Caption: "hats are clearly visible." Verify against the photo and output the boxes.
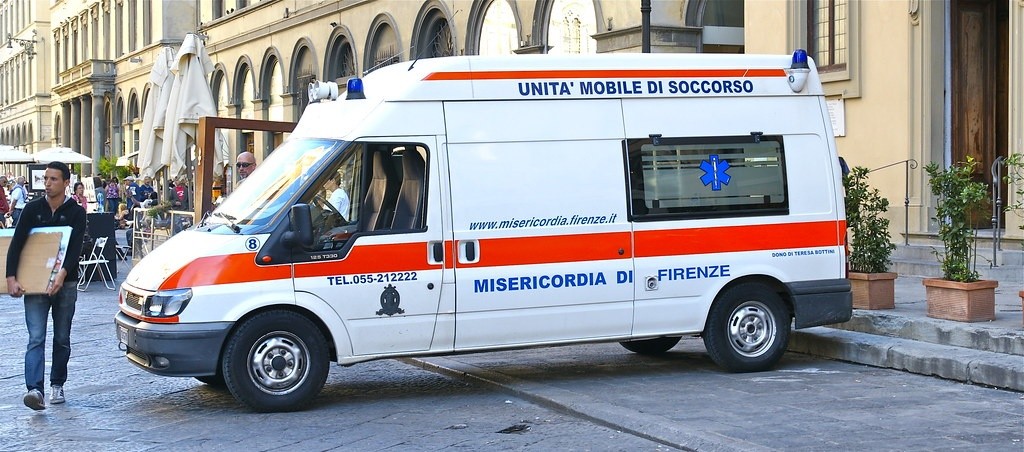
[123,176,134,180]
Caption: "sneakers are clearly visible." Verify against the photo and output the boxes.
[49,384,65,404]
[24,389,45,410]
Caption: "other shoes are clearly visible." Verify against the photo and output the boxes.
[123,252,132,256]
[119,255,128,261]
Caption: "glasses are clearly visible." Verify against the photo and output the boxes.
[236,162,255,168]
[2,181,8,183]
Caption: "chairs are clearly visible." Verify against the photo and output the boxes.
[76,237,116,291]
[358,149,400,231]
[389,147,425,231]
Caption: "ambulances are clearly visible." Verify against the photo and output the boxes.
[114,50,855,415]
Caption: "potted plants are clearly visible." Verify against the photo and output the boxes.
[999,153,1023,331]
[842,165,899,310]
[147,200,173,227]
[923,154,999,323]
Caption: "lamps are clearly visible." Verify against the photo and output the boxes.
[7,33,37,61]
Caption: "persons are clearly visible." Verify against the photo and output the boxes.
[4,159,87,410]
[70,173,193,232]
[0,175,28,229]
[322,172,350,224]
[234,150,257,191]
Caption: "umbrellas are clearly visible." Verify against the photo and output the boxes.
[159,33,226,213]
[136,44,178,206]
[29,143,94,165]
[0,147,36,163]
